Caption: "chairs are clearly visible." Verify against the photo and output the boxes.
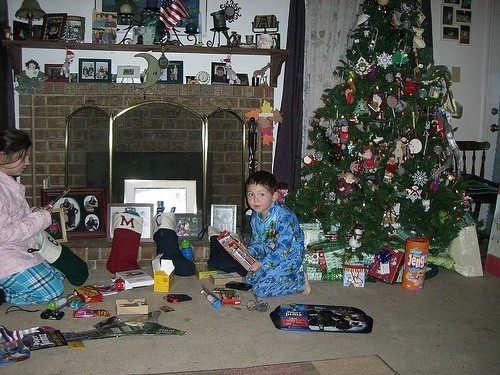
[455,141,499,273]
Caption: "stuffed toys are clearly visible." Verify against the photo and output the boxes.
[60,51,74,77]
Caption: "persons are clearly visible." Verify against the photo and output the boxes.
[49,64,108,78]
[0,127,64,308]
[172,65,178,80]
[46,24,74,39]
[92,13,116,44]
[25,60,39,78]
[50,196,99,235]
[215,66,226,81]
[240,170,311,298]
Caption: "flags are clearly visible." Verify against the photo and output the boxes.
[159,0,190,30]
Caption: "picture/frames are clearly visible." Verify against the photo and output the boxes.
[211,62,227,84]
[175,213,202,240]
[441,0,473,45]
[41,188,107,238]
[45,64,69,82]
[117,66,139,78]
[256,33,280,49]
[167,61,183,84]
[124,179,197,216]
[107,203,153,243]
[211,204,236,233]
[13,13,85,41]
[44,208,67,243]
[79,59,111,83]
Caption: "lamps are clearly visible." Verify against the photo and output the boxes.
[15,0,46,40]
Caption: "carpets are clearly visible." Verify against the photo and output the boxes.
[133,354,401,375]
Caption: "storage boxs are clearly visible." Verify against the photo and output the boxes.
[196,231,257,285]
[270,304,373,333]
[300,222,405,288]
[74,253,175,315]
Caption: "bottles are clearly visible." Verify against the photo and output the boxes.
[46,297,68,310]
[181,239,194,261]
